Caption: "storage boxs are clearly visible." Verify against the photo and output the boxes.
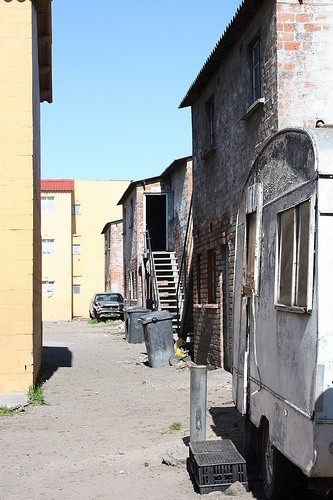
[185,437,251,495]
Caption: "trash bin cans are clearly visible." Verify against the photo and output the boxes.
[136,310,175,368]
[122,306,149,344]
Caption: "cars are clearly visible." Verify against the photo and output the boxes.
[89,292,125,323]
